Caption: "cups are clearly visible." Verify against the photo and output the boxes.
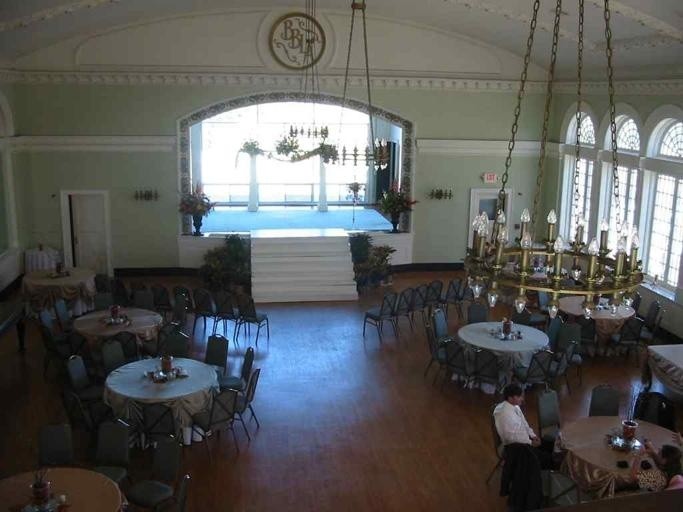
[21,481,70,512]
[143,357,186,387]
[487,322,523,341]
[100,302,130,328]
[602,417,642,457]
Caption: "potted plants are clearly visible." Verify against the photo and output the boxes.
[349,232,397,294]
[197,234,251,293]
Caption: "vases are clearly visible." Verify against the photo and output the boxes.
[192,214,204,236]
[389,211,401,233]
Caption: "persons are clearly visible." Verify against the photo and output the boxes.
[492,384,563,471]
[631,428,682,495]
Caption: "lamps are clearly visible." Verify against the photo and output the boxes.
[324,0,389,171]
[460,0,646,319]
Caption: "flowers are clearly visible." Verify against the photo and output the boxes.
[178,183,216,217]
[379,188,418,214]
